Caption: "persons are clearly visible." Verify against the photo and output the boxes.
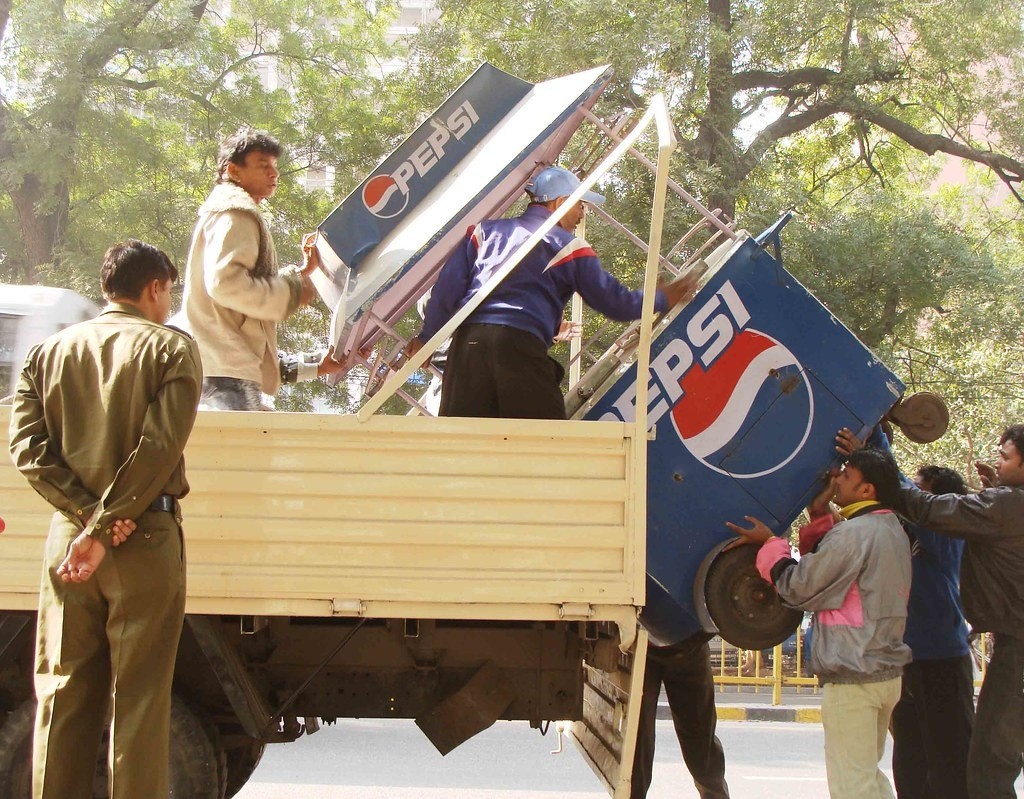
[164,128,373,412]
[632,641,732,799]
[721,420,1024,799]
[6,238,204,796]
[406,165,701,420]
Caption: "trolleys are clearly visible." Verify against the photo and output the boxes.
[310,62,949,650]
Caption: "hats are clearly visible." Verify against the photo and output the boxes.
[525,167,606,204]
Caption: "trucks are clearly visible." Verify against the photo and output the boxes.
[0,93,676,799]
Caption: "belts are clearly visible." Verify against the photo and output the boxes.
[147,495,175,512]
